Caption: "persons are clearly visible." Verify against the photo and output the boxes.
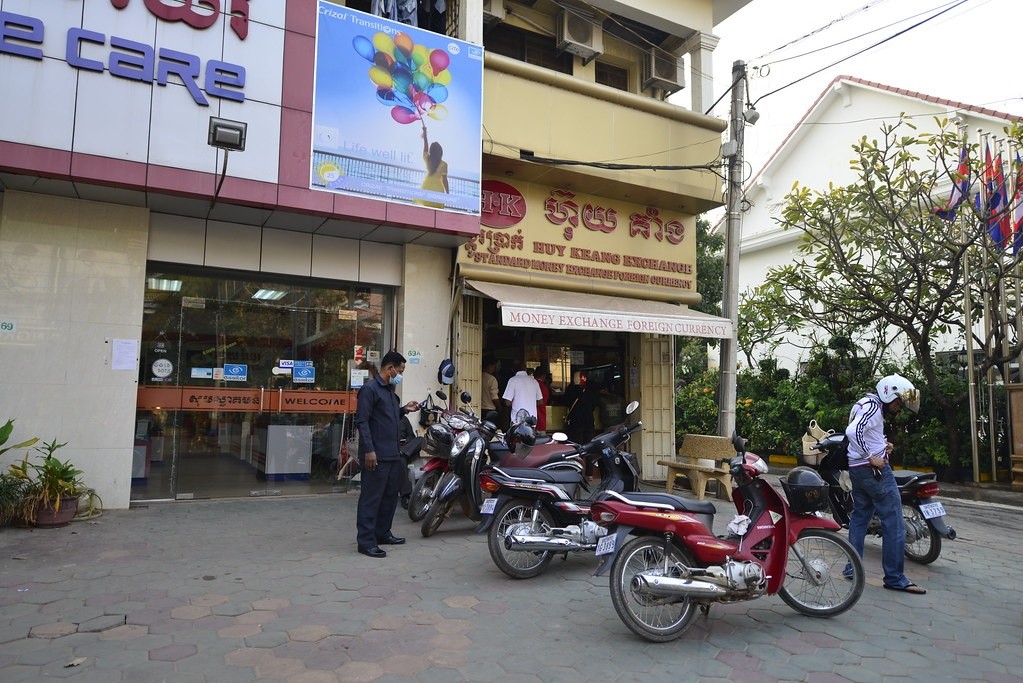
[415,126,450,210]
[841,374,926,594]
[480,355,552,435]
[356,352,427,558]
[598,382,628,451]
[562,370,597,481]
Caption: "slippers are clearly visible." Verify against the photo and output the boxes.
[848,576,853,580]
[883,583,926,594]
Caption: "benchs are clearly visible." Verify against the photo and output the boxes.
[657,435,748,502]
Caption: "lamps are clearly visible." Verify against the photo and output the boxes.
[208,117,248,213]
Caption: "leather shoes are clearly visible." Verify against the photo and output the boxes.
[358,545,386,557]
[378,535,406,544]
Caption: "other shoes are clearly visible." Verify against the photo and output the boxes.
[401,495,410,509]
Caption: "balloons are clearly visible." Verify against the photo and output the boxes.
[352,31,452,124]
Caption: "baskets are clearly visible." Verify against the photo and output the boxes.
[592,451,640,476]
[797,451,829,470]
[421,434,451,459]
[779,477,830,512]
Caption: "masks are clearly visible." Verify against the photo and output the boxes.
[389,367,403,385]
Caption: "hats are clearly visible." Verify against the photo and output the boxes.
[441,359,455,385]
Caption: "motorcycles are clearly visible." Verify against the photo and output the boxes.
[806,425,956,565]
[408,390,555,522]
[588,436,865,643]
[472,401,647,578]
[421,392,591,537]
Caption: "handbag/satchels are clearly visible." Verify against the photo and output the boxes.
[802,420,835,465]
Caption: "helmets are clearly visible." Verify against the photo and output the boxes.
[426,423,453,449]
[875,372,915,404]
[506,423,537,453]
[785,466,825,508]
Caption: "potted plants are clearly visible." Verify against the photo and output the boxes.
[19,437,103,527]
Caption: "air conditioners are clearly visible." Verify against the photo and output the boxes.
[556,10,604,63]
[483,0,505,25]
[643,48,686,94]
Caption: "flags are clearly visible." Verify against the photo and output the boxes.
[928,132,1023,254]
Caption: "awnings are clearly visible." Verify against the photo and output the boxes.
[466,279,733,340]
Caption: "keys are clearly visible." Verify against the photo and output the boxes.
[872,467,883,480]
